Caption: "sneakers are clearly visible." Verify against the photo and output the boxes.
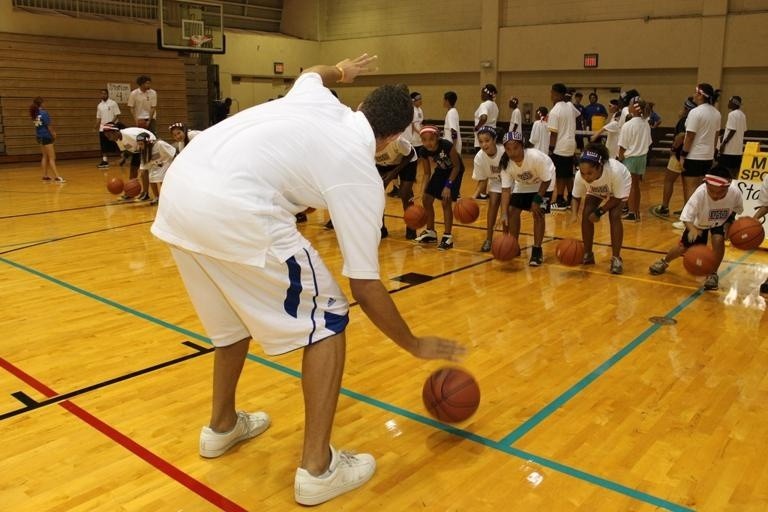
[566,201,572,209]
[382,227,388,237]
[436,235,454,251]
[549,199,567,210]
[760,278,768,294]
[648,257,670,276]
[293,443,378,507]
[198,410,271,459]
[120,159,125,166]
[40,176,54,182]
[703,271,719,290]
[55,177,66,182]
[515,246,521,257]
[296,213,307,223]
[414,229,437,243]
[655,206,670,216]
[622,209,628,213]
[621,213,636,222]
[386,185,401,198]
[609,256,624,274]
[528,246,543,266]
[481,240,492,252]
[97,160,109,167]
[117,192,158,206]
[322,219,333,230]
[671,220,686,230]
[476,192,488,201]
[406,226,417,240]
[582,251,595,265]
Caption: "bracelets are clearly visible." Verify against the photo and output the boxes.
[336,65,344,83]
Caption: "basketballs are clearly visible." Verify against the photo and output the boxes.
[454,197,479,223]
[729,216,764,249]
[492,234,518,261]
[556,239,584,265]
[684,245,716,276]
[404,204,427,229]
[125,180,141,197]
[422,369,480,423]
[107,178,124,194]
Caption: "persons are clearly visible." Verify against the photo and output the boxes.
[29,97,65,184]
[650,83,767,294]
[217,97,232,122]
[95,75,205,205]
[328,82,660,275]
[150,53,465,508]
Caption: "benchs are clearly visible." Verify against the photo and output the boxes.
[417,114,768,166]
